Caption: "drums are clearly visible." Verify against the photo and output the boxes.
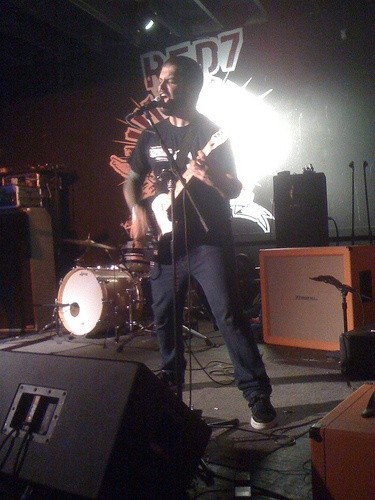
[119,240,154,271]
[56,264,145,337]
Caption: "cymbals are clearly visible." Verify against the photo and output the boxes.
[59,236,118,251]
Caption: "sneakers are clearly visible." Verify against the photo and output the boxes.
[249,399,276,429]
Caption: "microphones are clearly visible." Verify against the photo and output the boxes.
[128,95,166,119]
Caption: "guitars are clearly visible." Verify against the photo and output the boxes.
[148,127,230,243]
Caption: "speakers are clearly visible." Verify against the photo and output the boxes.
[0,351,212,500]
[272,172,329,248]
[309,383,375,500]
[259,244,375,350]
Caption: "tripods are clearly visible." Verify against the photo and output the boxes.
[118,288,144,332]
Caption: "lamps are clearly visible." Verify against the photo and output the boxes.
[139,16,156,31]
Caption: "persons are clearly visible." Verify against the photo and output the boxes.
[123,56,278,430]
